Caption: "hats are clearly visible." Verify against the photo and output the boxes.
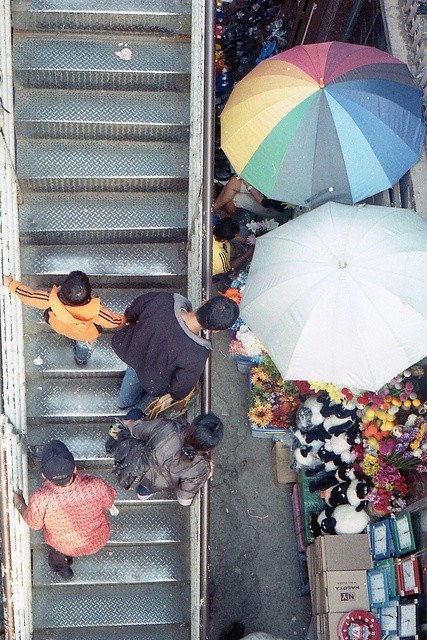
[41,440,74,484]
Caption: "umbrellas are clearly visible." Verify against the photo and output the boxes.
[238,201,426,396]
[218,40,426,209]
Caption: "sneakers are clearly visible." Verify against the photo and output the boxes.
[136,492,159,500]
[220,621,244,640]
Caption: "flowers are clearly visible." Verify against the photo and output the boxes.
[224,272,248,305]
[229,326,266,360]
[248,360,310,429]
[246,219,279,246]
[312,364,426,504]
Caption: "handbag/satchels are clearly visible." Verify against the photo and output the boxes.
[113,438,153,491]
[142,386,199,420]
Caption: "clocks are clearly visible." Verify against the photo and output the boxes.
[381,595,400,635]
[398,605,421,637]
[367,570,389,608]
[337,610,381,640]
[400,555,422,596]
[389,520,399,557]
[371,520,390,560]
[375,559,396,598]
[394,512,417,554]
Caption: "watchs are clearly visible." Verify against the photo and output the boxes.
[16,499,24,511]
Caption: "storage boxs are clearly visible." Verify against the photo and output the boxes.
[292,483,307,553]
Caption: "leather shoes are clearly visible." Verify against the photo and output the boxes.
[59,566,73,581]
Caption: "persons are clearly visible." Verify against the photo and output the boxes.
[213,176,290,218]
[121,408,223,506]
[112,291,239,413]
[3,271,126,365]
[220,621,275,639]
[13,440,117,581]
[213,217,255,293]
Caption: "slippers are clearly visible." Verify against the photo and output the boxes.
[254,40,275,64]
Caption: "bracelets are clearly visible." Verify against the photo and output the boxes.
[248,186,252,191]
[241,254,248,260]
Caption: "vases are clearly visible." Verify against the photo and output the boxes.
[248,355,311,440]
[236,356,258,374]
